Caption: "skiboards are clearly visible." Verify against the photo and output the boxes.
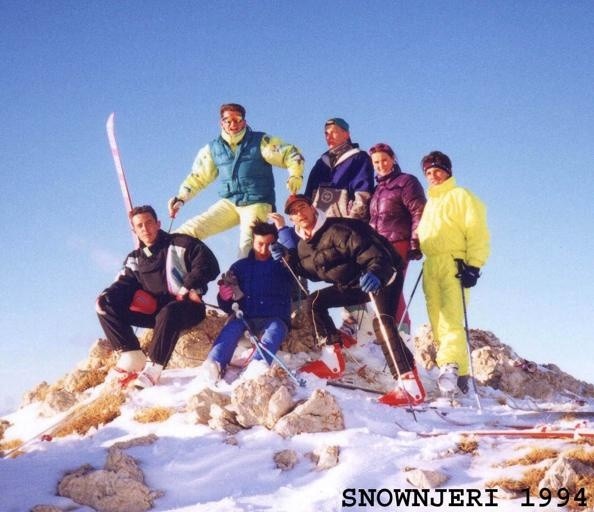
[395,400,594,439]
[105,111,140,251]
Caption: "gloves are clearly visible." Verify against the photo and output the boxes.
[406,249,423,260]
[218,270,244,301]
[359,271,382,296]
[168,194,187,217]
[176,285,190,302]
[286,176,304,195]
[268,242,291,263]
[95,288,115,316]
[462,266,481,288]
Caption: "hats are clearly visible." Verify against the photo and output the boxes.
[423,151,452,176]
[324,117,352,145]
[284,193,313,215]
[370,142,395,161]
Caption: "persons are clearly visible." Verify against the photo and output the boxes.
[282,194,427,408]
[164,103,306,260]
[301,118,375,348]
[411,151,490,396]
[93,204,221,392]
[362,142,425,335]
[190,210,302,386]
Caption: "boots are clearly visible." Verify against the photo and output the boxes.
[295,343,347,384]
[351,305,378,338]
[379,367,425,408]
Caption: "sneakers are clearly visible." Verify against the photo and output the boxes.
[231,360,272,391]
[192,360,227,391]
[100,366,153,389]
[437,361,458,393]
